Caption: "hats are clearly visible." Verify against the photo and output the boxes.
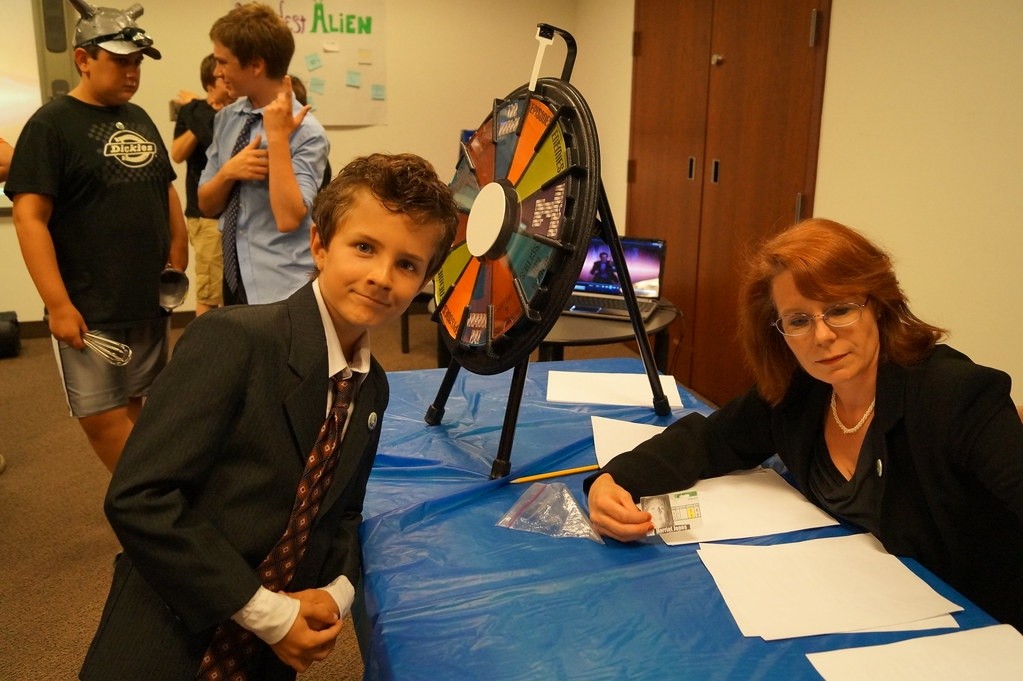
[70,0,162,62]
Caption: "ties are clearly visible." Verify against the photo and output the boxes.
[197,364,357,681]
[222,114,260,293]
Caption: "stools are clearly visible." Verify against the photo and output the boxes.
[401,280,435,354]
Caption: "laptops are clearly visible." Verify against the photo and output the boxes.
[561,236,666,320]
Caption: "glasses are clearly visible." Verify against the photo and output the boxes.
[769,292,870,336]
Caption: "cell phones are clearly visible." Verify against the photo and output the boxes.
[570,304,602,313]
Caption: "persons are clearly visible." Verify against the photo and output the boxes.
[584,219,1023,631]
[2,0,189,471]
[590,253,619,285]
[171,55,237,316]
[640,495,674,529]
[197,3,330,306]
[290,76,332,190]
[0,138,16,473]
[76,153,460,681]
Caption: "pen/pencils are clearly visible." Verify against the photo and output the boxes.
[510,465,599,483]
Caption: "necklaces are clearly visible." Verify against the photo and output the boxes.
[831,390,876,433]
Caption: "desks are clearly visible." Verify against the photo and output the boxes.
[352,358,997,681]
[534,293,678,375]
